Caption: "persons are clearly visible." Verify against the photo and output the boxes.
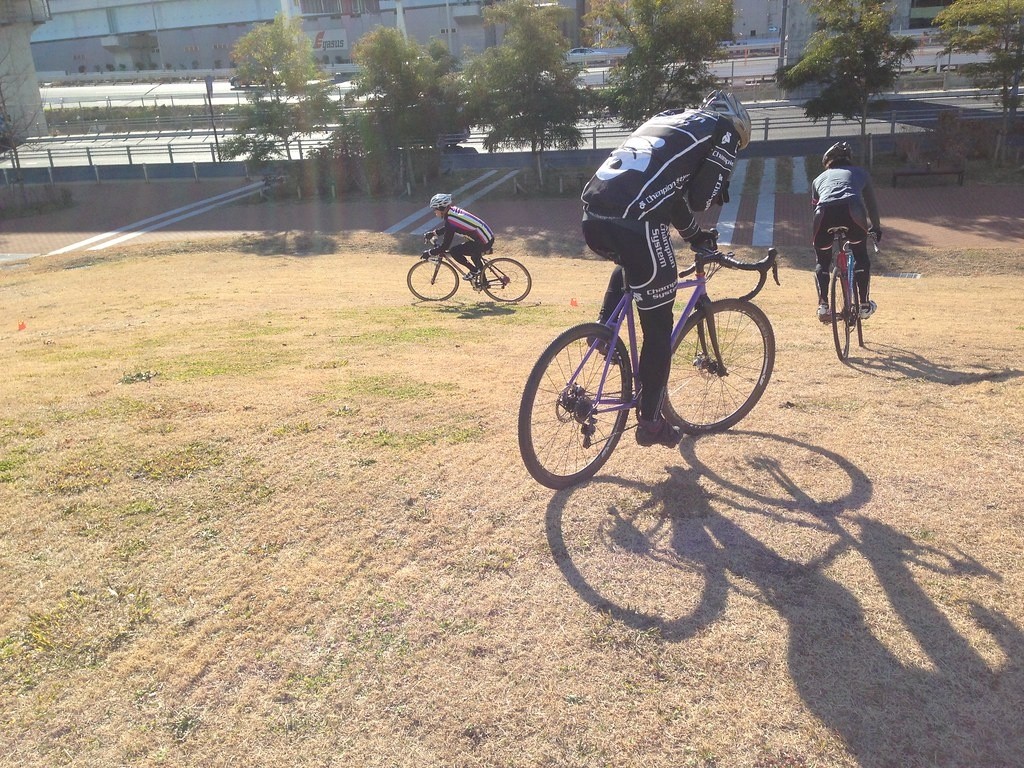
[420,193,495,289]
[580,88,753,449]
[811,142,883,322]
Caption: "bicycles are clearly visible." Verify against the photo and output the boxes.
[407,232,532,305]
[515,233,779,490]
[829,224,887,362]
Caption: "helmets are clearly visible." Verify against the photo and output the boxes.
[699,89,751,150]
[822,141,852,166]
[430,193,452,209]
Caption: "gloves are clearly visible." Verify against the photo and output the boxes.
[420,250,430,259]
[423,231,434,244]
[684,228,717,252]
[867,226,882,242]
[717,181,730,207]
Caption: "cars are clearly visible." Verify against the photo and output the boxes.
[565,46,608,66]
[229,68,281,90]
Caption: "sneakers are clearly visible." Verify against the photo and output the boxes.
[586,320,618,365]
[636,416,683,449]
[817,303,830,322]
[859,300,878,319]
[462,269,481,280]
